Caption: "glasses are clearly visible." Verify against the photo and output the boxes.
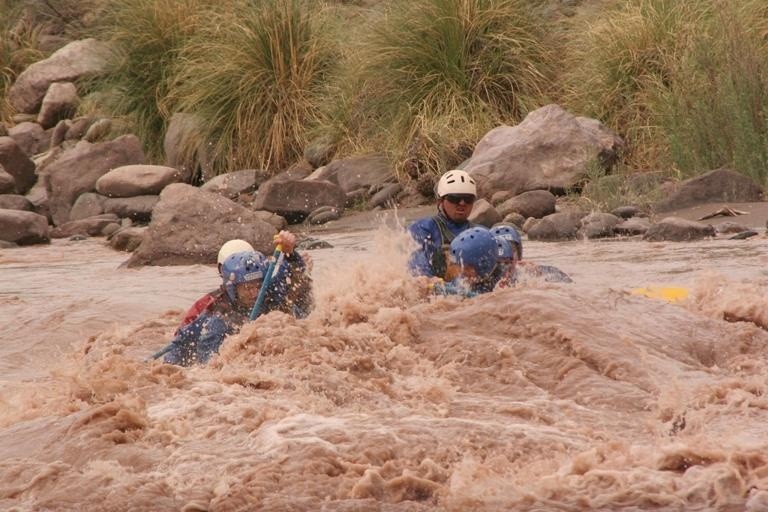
[444,194,475,204]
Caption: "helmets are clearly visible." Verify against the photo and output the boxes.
[448,225,522,278]
[437,170,477,199]
[217,239,270,303]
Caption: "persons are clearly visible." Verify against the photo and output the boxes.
[404,167,572,298]
[163,230,312,370]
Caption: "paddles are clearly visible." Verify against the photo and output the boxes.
[631,286,693,303]
